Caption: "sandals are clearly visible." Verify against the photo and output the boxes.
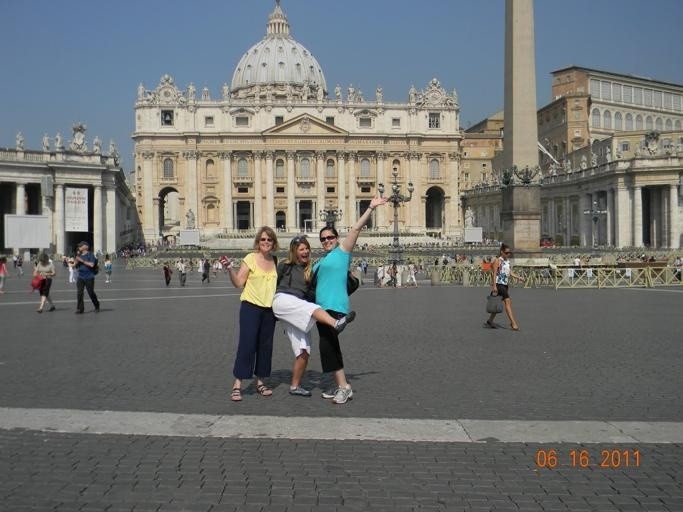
[230,383,242,401]
[255,383,272,396]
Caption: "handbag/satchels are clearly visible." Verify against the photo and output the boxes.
[486,291,503,313]
[91,259,98,275]
[30,275,43,289]
[305,269,359,301]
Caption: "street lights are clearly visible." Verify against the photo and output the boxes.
[375,165,414,261]
[583,199,608,247]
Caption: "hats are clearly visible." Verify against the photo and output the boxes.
[75,241,88,246]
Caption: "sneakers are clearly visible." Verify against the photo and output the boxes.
[35,308,42,313]
[510,323,520,331]
[321,379,353,404]
[45,305,55,311]
[483,318,499,330]
[73,308,84,314]
[94,306,99,313]
[330,311,355,334]
[288,384,311,396]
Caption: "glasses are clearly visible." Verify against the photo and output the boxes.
[502,251,512,255]
[291,236,306,246]
[319,236,336,242]
[259,238,272,242]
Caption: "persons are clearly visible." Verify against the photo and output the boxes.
[137,84,147,102]
[14,133,26,149]
[222,85,230,101]
[68,241,100,314]
[42,134,62,149]
[0,241,683,294]
[252,85,384,105]
[33,254,56,313]
[550,146,645,175]
[409,86,419,106]
[272,235,356,396]
[185,85,196,100]
[94,138,103,151]
[219,226,280,401]
[486,243,525,331]
[309,195,388,404]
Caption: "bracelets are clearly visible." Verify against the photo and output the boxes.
[368,205,374,210]
[518,277,521,281]
